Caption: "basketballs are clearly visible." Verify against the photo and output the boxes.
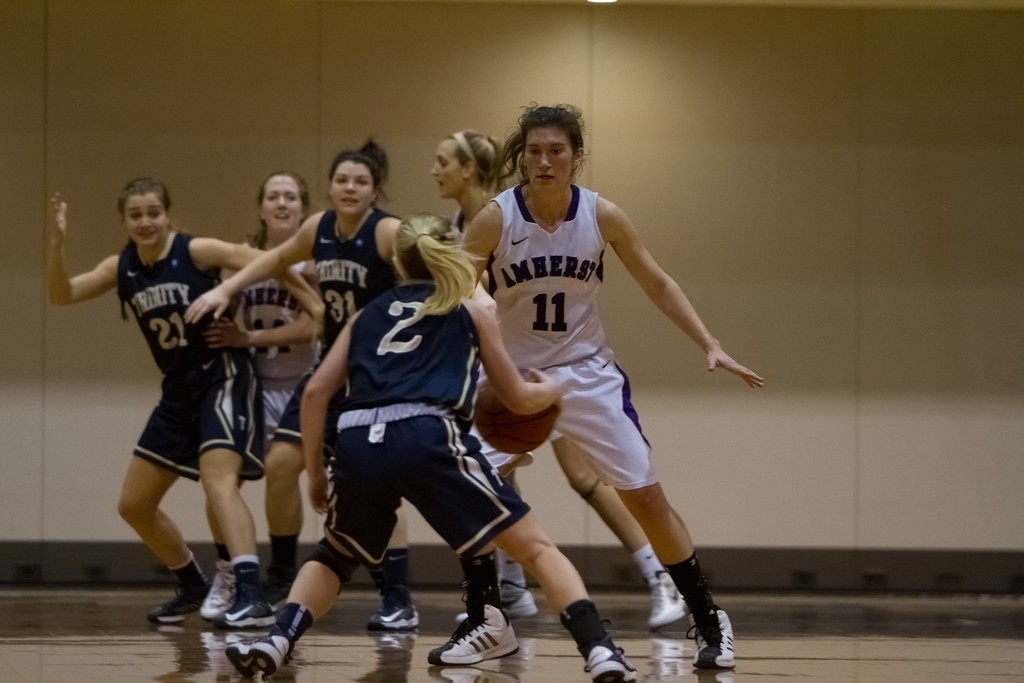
[473,369,559,453]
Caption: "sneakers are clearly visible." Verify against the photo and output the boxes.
[366,584,419,631]
[647,570,690,630]
[147,550,211,624]
[428,665,520,683]
[686,605,736,669]
[225,625,290,681]
[224,562,277,629]
[428,580,520,665]
[260,566,295,613]
[455,577,538,622]
[200,558,237,622]
[577,631,639,683]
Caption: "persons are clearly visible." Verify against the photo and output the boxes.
[425,107,765,671]
[31,176,326,635]
[198,139,638,683]
[428,128,692,631]
